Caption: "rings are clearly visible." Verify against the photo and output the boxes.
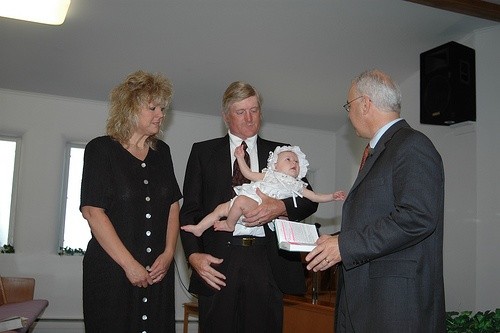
[325,259,330,263]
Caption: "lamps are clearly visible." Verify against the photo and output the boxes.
[0,0,73,25]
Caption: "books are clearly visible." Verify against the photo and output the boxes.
[273,219,319,252]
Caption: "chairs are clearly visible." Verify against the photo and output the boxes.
[0,276,48,333]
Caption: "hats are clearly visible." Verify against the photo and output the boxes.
[267,145,309,180]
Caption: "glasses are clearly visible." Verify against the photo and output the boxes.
[344,95,371,112]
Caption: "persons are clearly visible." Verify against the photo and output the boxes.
[306,71,448,333]
[181,144,346,238]
[179,82,318,333]
[79,71,183,333]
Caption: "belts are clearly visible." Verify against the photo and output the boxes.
[234,237,266,246]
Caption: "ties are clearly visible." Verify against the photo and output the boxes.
[359,142,370,173]
[232,141,251,185]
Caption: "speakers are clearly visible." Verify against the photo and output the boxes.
[420,41,476,125]
